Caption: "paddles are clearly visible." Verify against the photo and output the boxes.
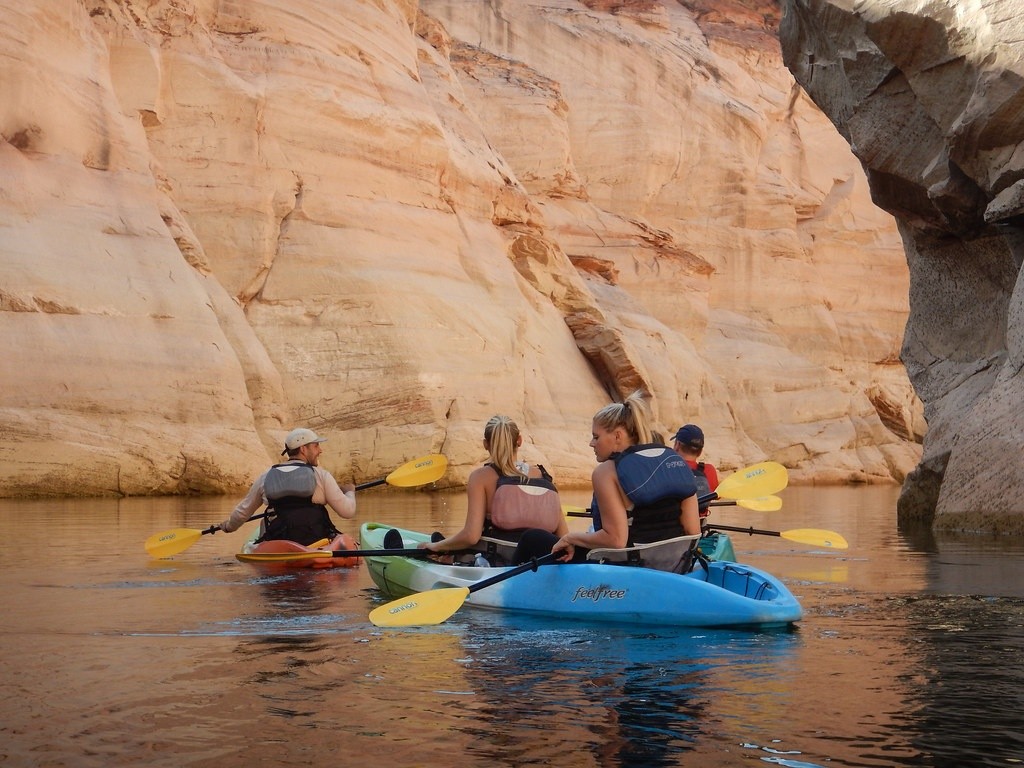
[143,453,450,560]
[560,494,784,520]
[368,458,790,628]
[234,547,476,563]
[560,503,850,551]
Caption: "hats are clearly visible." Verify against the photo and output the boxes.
[281,428,329,456]
[670,424,705,448]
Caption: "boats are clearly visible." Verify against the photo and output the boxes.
[236,519,366,567]
[360,521,804,631]
[695,516,736,562]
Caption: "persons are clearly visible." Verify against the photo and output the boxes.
[669,424,718,526]
[420,416,568,566]
[218,427,356,548]
[550,391,701,563]
